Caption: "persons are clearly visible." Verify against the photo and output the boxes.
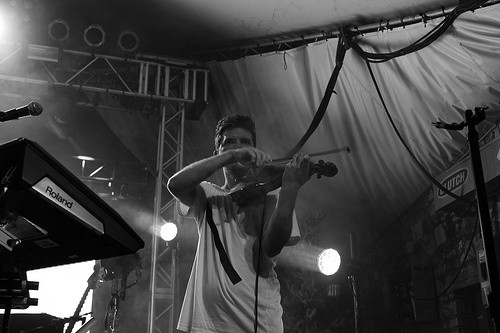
[165,114,317,333]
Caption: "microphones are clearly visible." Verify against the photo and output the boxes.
[0,100,43,122]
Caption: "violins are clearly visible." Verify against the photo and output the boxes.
[230,155,338,207]
